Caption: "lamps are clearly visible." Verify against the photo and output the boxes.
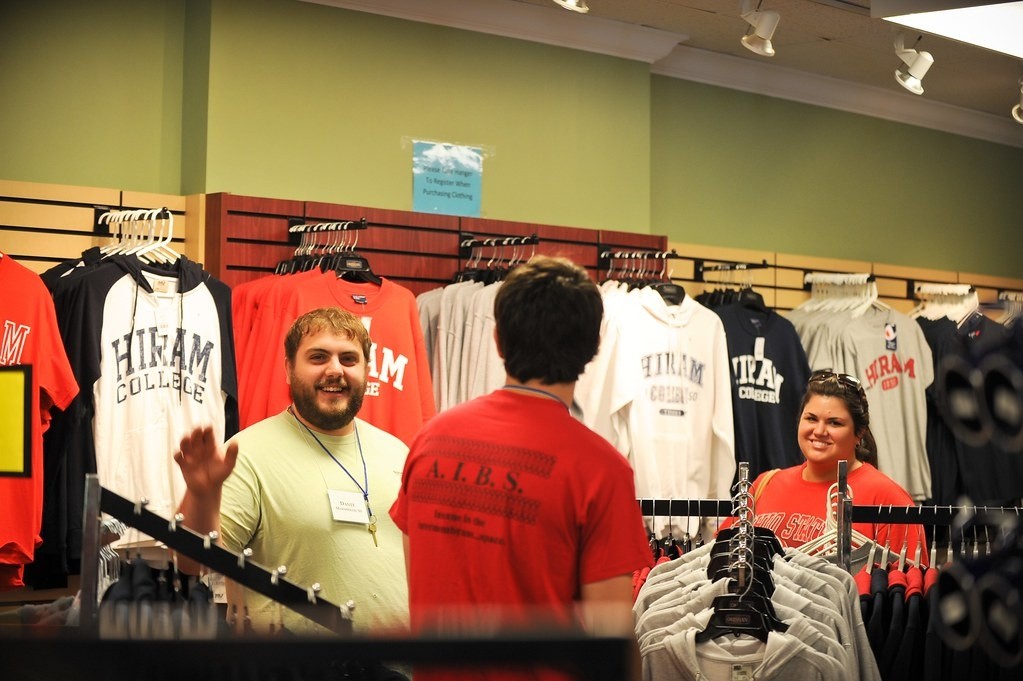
[552,0,590,14]
[893,30,935,95]
[740,0,781,57]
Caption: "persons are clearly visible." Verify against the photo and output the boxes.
[713,371,931,570]
[386,254,656,681]
[166,307,412,639]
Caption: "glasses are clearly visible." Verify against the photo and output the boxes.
[809,371,864,401]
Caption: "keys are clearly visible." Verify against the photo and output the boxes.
[369,515,378,547]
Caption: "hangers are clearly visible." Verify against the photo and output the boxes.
[60,207,183,278]
[66,518,297,638]
[995,290,1023,328]
[797,272,891,317]
[597,250,686,306]
[443,236,536,286]
[693,262,767,313]
[270,220,382,288]
[907,282,983,329]
[637,477,1023,644]
